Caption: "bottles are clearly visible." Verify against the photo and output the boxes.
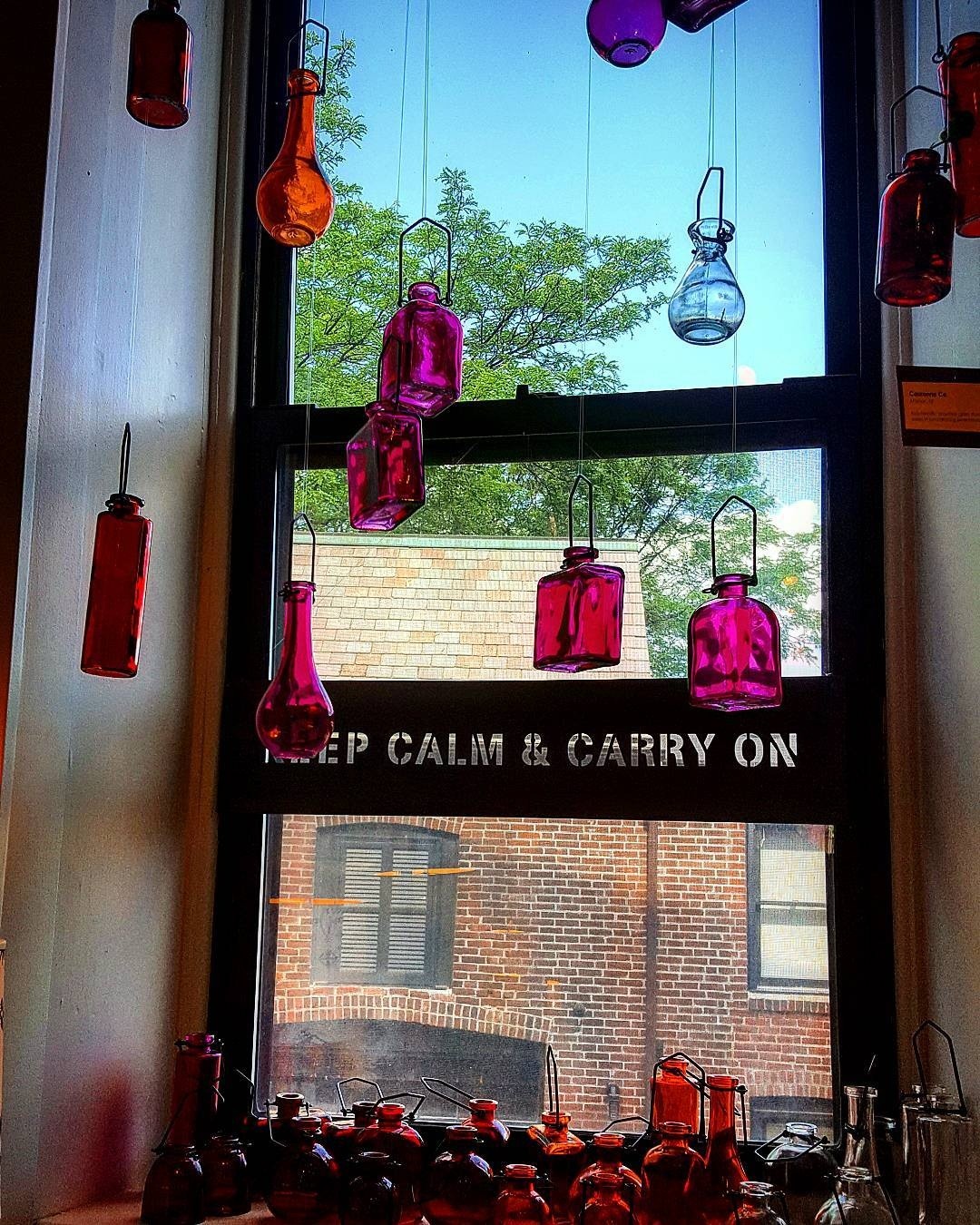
[381,280,464,419]
[686,573,783,712]
[667,217,746,345]
[873,148,958,308]
[938,32,980,240]
[80,493,154,679]
[255,68,337,249]
[125,0,197,129]
[255,580,336,759]
[141,1017,980,1225]
[533,547,626,674]
[346,401,427,532]
[585,0,748,69]
